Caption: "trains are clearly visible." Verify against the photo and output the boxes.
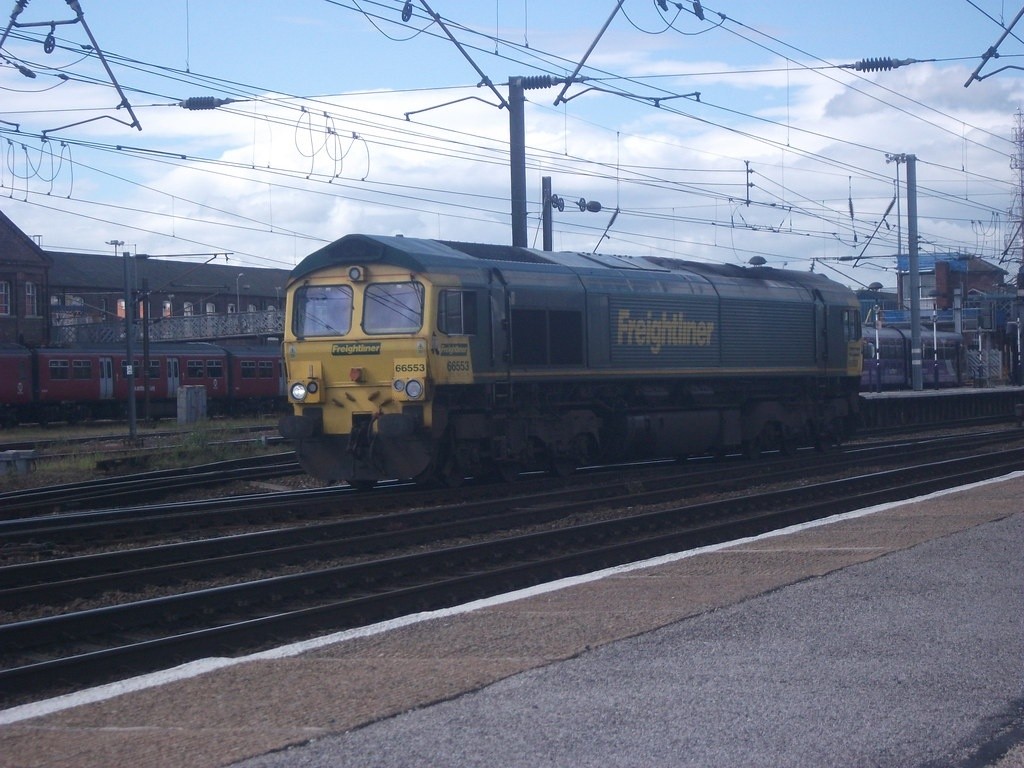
[267,230,866,472]
[1,320,970,419]
